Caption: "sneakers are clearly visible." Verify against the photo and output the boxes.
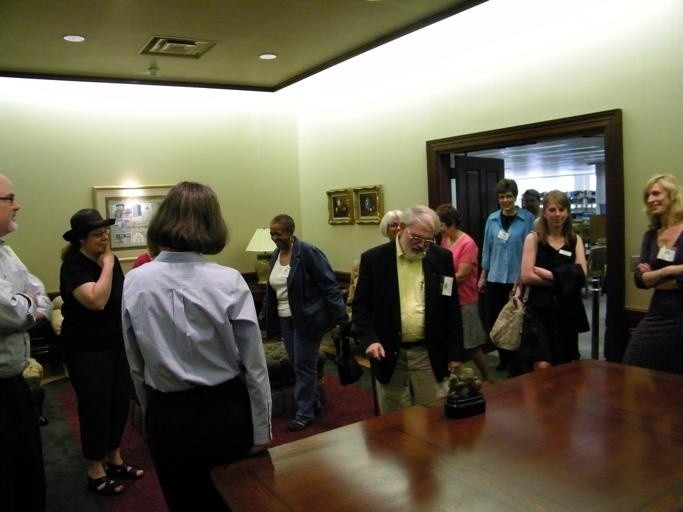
[287,415,313,432]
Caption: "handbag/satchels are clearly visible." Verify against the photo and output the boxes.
[334,337,363,386]
[489,293,528,350]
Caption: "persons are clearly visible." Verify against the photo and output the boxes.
[623,174,683,377]
[379,210,404,243]
[121,182,273,512]
[361,206,465,416]
[477,179,538,372]
[59,209,145,495]
[1,175,55,512]
[436,203,494,384]
[521,189,587,373]
[258,213,348,432]
[524,189,543,220]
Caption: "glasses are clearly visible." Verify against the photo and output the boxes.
[407,225,436,248]
[88,229,110,238]
[1,195,15,203]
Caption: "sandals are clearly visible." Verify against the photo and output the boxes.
[104,457,145,480]
[87,476,126,494]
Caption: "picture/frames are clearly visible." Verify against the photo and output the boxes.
[326,188,354,225]
[352,184,383,225]
[92,184,177,262]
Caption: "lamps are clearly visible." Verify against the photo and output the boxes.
[245,229,277,285]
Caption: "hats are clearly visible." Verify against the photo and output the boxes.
[64,209,116,241]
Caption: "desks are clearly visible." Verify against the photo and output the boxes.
[209,359,683,512]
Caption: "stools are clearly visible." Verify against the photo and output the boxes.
[263,341,327,419]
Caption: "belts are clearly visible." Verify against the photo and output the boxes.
[400,341,425,350]
